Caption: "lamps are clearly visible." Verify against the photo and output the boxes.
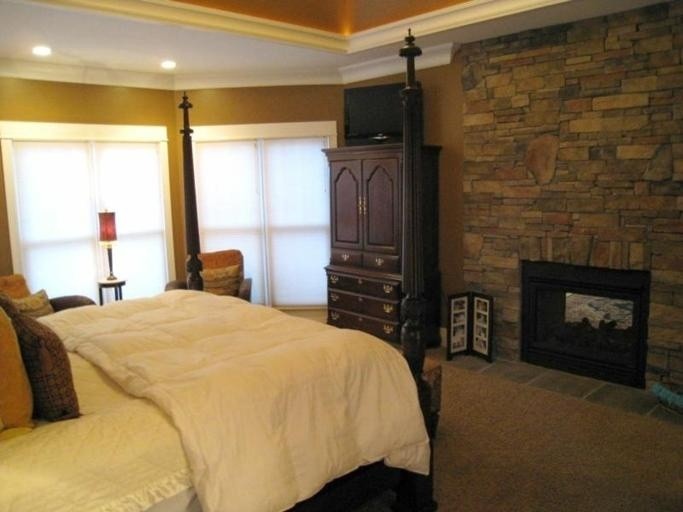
[95,208,117,280]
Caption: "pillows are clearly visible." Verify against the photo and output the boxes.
[0,308,37,428]
[0,297,81,422]
[11,289,55,319]
[200,265,240,295]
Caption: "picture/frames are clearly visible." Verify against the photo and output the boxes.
[450,293,492,360]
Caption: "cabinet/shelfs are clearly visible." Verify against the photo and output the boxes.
[321,142,441,345]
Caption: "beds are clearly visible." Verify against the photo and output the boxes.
[0,281,430,512]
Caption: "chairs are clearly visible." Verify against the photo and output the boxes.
[165,249,252,300]
[0,274,96,321]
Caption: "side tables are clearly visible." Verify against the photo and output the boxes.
[100,282,126,305]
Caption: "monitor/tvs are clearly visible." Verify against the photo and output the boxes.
[343,83,423,146]
